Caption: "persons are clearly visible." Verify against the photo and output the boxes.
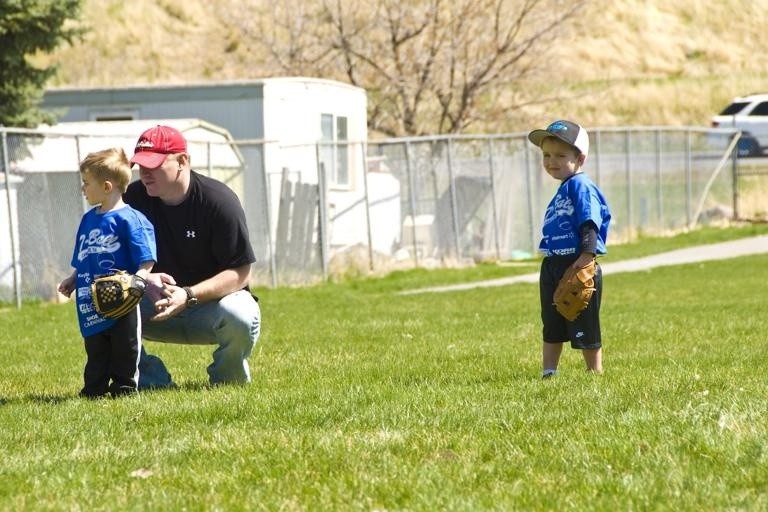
[525,120,612,380]
[121,123,261,393]
[55,145,157,398]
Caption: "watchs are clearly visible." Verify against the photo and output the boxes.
[183,284,197,311]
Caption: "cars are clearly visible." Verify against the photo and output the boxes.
[707,94,768,157]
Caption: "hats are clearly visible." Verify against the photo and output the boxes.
[129,125,188,170]
[527,119,590,161]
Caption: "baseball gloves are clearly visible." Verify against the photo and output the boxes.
[550,258,597,323]
[90,269,147,320]
